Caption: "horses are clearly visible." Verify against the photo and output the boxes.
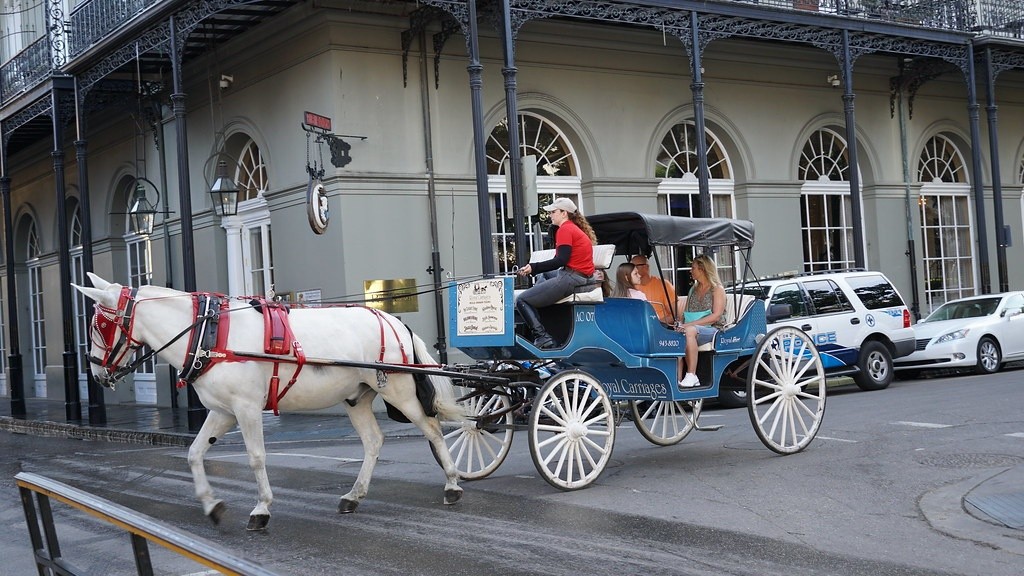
[70,272,473,530]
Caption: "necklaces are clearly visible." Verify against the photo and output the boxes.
[516,197,596,348]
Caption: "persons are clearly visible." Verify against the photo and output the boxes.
[678,255,727,388]
[594,269,610,297]
[612,263,646,300]
[628,255,675,323]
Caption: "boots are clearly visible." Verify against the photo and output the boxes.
[516,299,554,349]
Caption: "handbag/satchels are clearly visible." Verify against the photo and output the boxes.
[683,310,712,328]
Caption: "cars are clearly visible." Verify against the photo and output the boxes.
[890,289,1024,376]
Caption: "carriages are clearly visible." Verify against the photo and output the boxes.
[70,211,826,531]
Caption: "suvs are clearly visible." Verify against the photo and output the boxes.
[718,267,916,409]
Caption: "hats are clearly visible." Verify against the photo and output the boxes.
[543,198,577,216]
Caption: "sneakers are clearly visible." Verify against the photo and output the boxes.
[680,372,700,387]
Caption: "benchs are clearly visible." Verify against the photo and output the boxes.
[675,294,755,390]
[512,244,616,349]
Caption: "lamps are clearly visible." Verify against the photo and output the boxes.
[203,16,243,217]
[123,61,160,235]
